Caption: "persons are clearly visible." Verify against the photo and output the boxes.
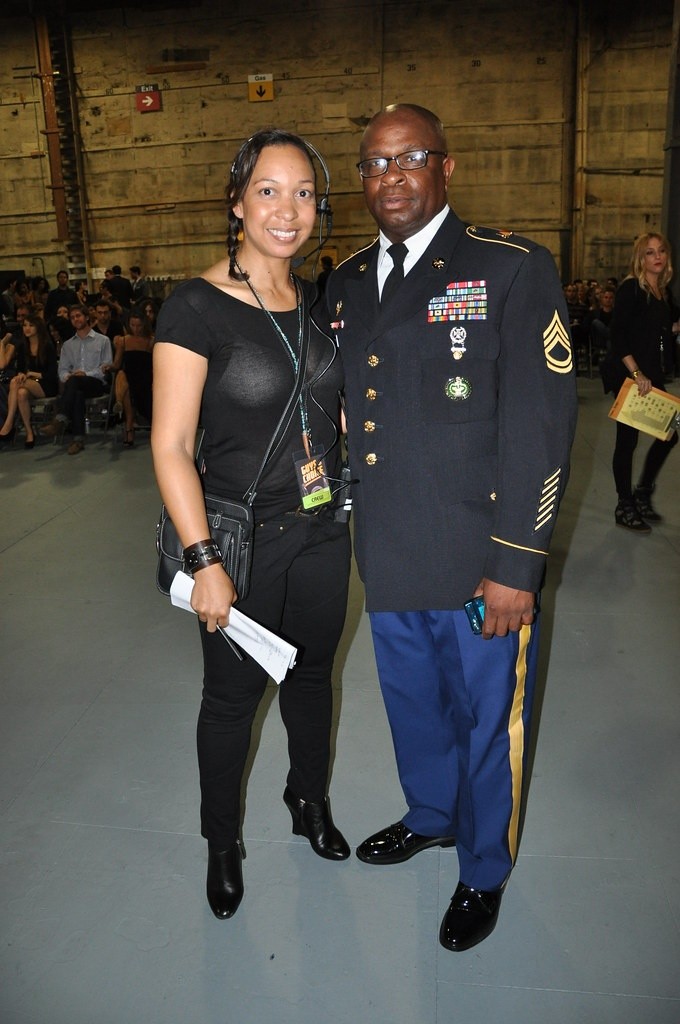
[151,128,352,920]
[316,256,338,290]
[604,233,680,534]
[324,105,579,953]
[563,278,618,375]
[1,265,162,453]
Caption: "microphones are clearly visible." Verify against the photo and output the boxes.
[291,213,333,269]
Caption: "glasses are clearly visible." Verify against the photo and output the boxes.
[355,150,446,178]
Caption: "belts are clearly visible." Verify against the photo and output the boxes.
[285,502,324,518]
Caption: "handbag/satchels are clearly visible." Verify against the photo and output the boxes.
[155,492,256,603]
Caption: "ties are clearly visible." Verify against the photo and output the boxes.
[380,243,409,307]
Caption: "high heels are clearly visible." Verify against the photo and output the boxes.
[113,400,124,419]
[282,785,351,861]
[0,427,16,444]
[123,428,135,448]
[25,434,36,448]
[206,838,248,920]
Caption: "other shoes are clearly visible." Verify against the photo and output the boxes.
[68,441,85,454]
[633,486,663,524]
[39,420,64,436]
[615,499,652,534]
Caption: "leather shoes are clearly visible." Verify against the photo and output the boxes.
[356,821,456,865]
[439,869,511,952]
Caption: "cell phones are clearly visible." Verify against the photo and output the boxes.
[464,595,539,636]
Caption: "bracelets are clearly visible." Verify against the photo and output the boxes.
[630,370,644,379]
[183,539,222,573]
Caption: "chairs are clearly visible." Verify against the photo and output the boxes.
[59,369,151,444]
[13,359,60,444]
